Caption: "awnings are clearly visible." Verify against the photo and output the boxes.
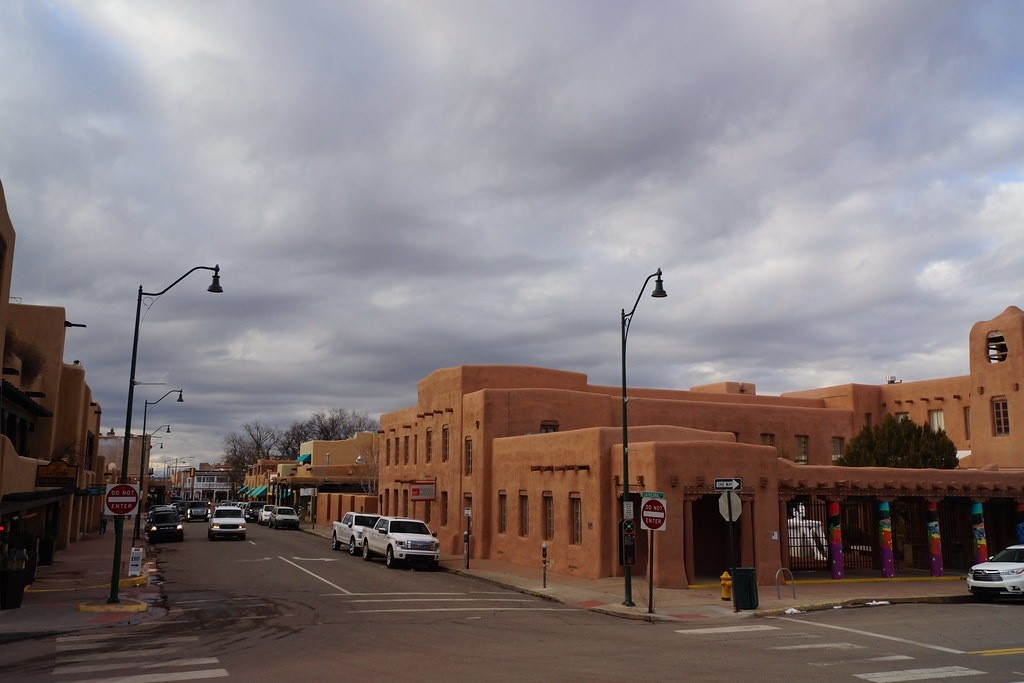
[250,486,263,496]
[297,454,311,463]
[256,486,267,497]
[238,486,248,493]
[246,488,256,495]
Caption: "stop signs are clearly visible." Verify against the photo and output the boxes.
[105,486,140,515]
[641,498,666,529]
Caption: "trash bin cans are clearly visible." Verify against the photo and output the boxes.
[731,567,759,610]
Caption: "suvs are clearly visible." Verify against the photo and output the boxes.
[269,506,300,528]
[187,501,210,522]
[258,505,277,526]
[147,508,185,543]
[208,506,247,540]
[966,544,1024,599]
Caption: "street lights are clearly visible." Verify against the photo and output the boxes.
[174,456,196,488]
[143,424,171,465]
[107,263,224,604]
[621,267,668,606]
[136,387,184,541]
[164,458,185,490]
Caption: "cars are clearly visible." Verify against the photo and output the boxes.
[144,499,187,533]
[219,499,267,522]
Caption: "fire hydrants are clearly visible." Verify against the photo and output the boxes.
[720,571,732,600]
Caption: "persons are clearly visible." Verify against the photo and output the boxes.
[98,508,108,535]
[284,502,299,513]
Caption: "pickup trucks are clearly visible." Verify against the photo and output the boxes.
[329,512,383,555]
[362,516,440,570]
